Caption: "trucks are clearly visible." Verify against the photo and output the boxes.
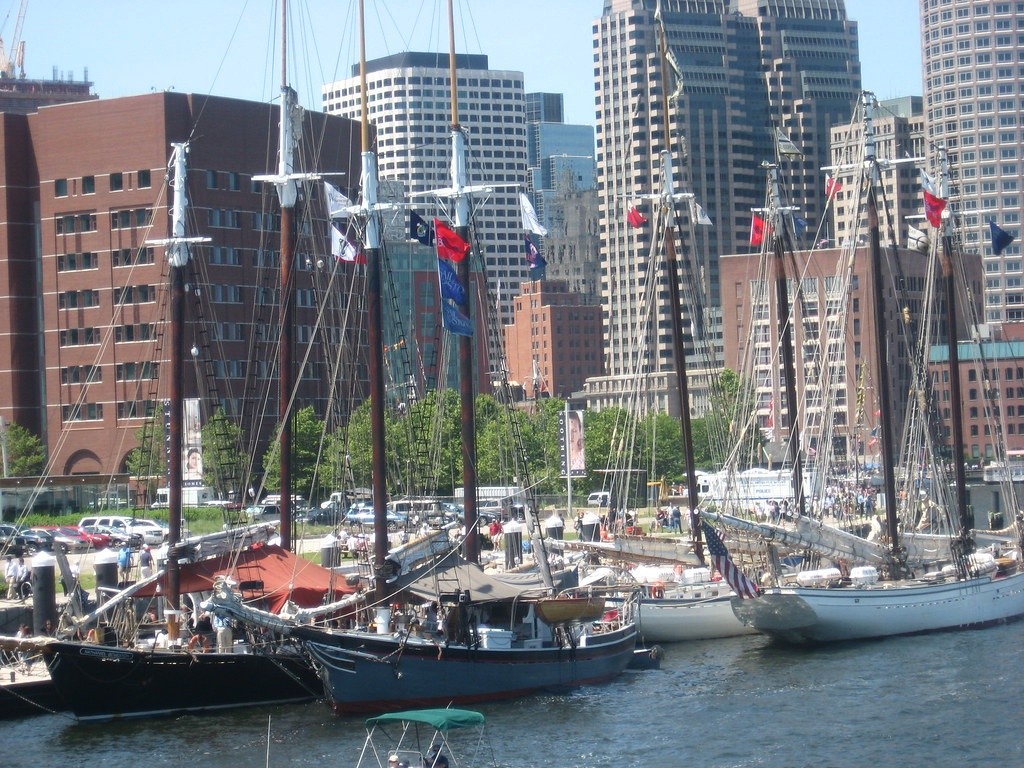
[150,483,214,507]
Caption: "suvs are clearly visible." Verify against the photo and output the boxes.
[0,524,51,555]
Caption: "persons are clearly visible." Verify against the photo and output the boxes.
[116,542,153,581]
[398,525,410,545]
[490,517,504,552]
[3,553,28,600]
[388,755,413,768]
[600,505,684,534]
[752,479,878,525]
[59,561,80,596]
[569,411,585,470]
[334,526,370,558]
[422,744,448,768]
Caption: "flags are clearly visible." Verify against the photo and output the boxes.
[774,128,806,163]
[792,211,808,234]
[990,220,1014,255]
[920,167,947,228]
[824,175,841,199]
[409,211,473,335]
[322,180,367,266]
[688,199,713,226]
[700,522,761,600]
[751,213,772,246]
[518,191,549,281]
[628,200,647,228]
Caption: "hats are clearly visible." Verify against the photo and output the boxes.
[388,755,399,762]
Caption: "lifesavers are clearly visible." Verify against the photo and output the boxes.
[653,582,665,599]
[189,634,209,653]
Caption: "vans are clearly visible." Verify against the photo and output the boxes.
[385,499,447,520]
[586,491,610,508]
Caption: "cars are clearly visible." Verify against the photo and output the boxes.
[82,525,146,552]
[31,524,114,549]
[301,508,346,527]
[230,504,284,529]
[19,527,91,555]
[345,504,410,534]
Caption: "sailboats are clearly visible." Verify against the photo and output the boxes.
[0,0,1024,723]
